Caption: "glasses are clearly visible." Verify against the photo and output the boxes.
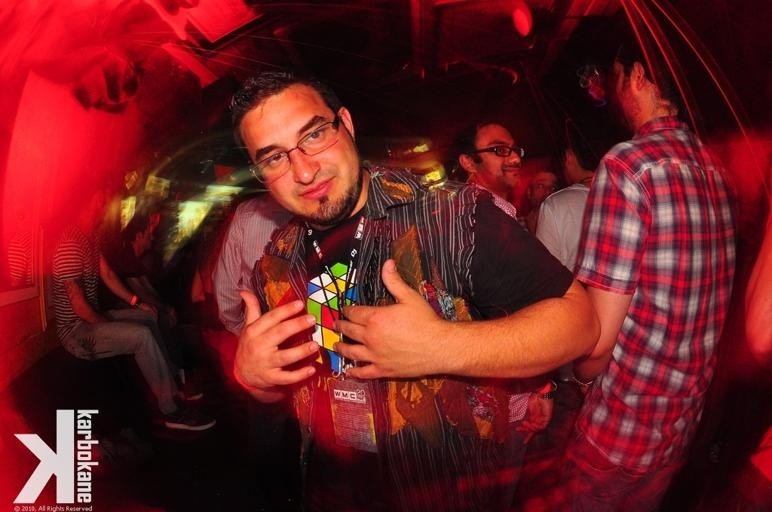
[248,114,340,184]
[471,145,525,158]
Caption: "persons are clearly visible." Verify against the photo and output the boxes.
[48,28,772,512]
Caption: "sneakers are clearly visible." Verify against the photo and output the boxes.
[173,382,203,401]
[165,407,217,430]
